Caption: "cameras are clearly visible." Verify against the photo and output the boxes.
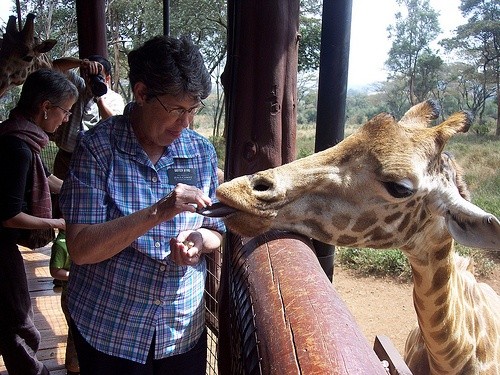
[84,67,107,97]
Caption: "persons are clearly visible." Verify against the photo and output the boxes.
[59,35,227,375]
[50,227,81,375]
[0,70,79,375]
[52,55,125,219]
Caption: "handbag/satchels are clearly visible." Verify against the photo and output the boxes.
[16,226,56,251]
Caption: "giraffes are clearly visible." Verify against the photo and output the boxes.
[0,13,58,100]
[216,97,500,375]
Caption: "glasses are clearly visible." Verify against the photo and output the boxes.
[152,94,207,116]
[52,105,73,116]
